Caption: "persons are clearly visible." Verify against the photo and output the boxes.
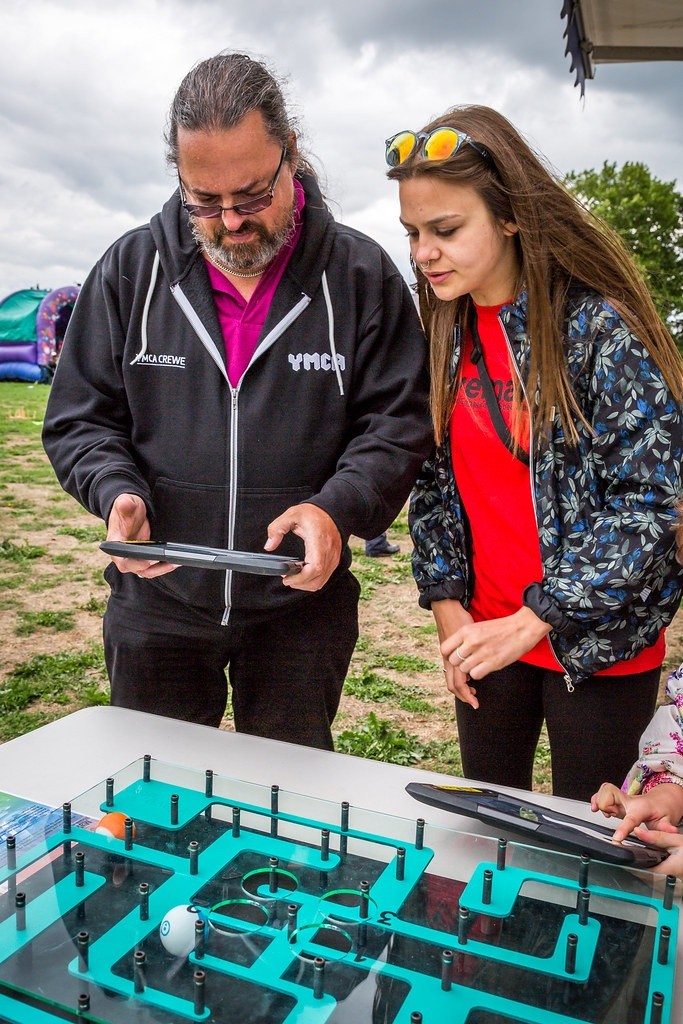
[591,662,683,896]
[387,103,683,804]
[40,47,432,753]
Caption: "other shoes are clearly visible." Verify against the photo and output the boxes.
[373,543,400,557]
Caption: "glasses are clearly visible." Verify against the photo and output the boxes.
[385,125,494,164]
[178,145,285,218]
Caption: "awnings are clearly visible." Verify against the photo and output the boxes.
[560,0,683,109]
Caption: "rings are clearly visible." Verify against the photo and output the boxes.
[137,570,144,578]
[442,669,447,673]
[457,647,466,660]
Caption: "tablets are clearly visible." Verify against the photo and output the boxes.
[405,782,671,869]
[99,539,306,576]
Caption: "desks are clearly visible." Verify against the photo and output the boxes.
[0,702,683,1021]
